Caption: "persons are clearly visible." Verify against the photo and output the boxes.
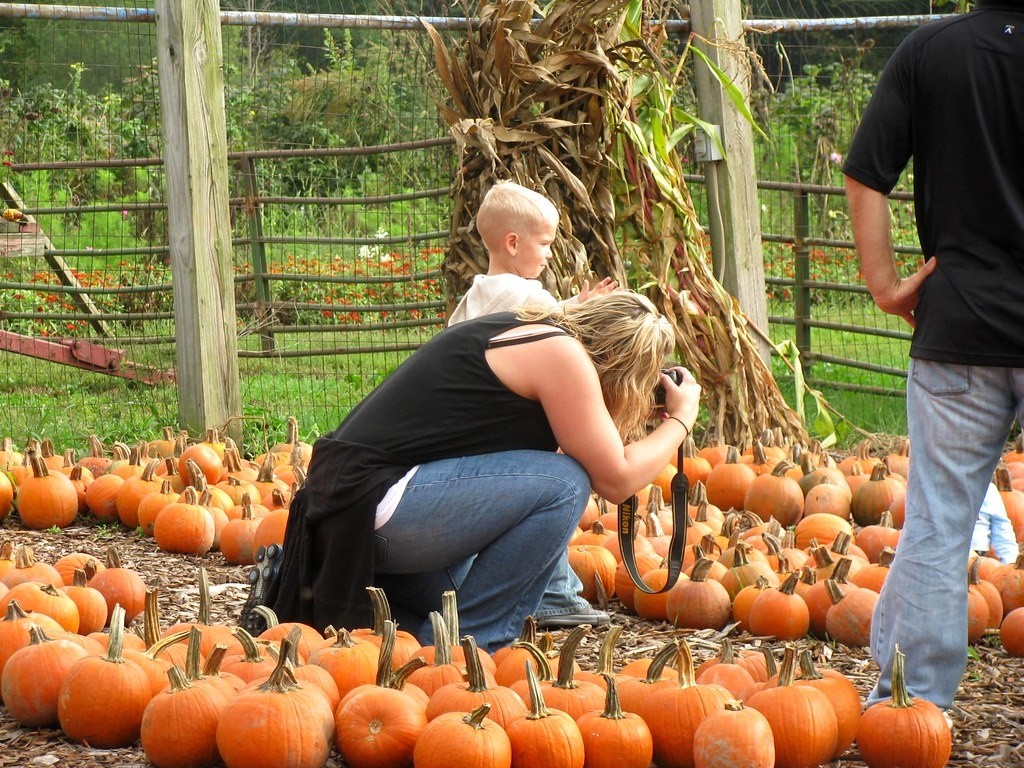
[237,292,702,656]
[970,482,1019,567]
[446,180,621,625]
[842,0,1024,714]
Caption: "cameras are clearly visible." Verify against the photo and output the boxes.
[650,369,683,412]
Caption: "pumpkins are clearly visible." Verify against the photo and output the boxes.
[0,414,1024,764]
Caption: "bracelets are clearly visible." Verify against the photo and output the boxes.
[669,416,689,436]
